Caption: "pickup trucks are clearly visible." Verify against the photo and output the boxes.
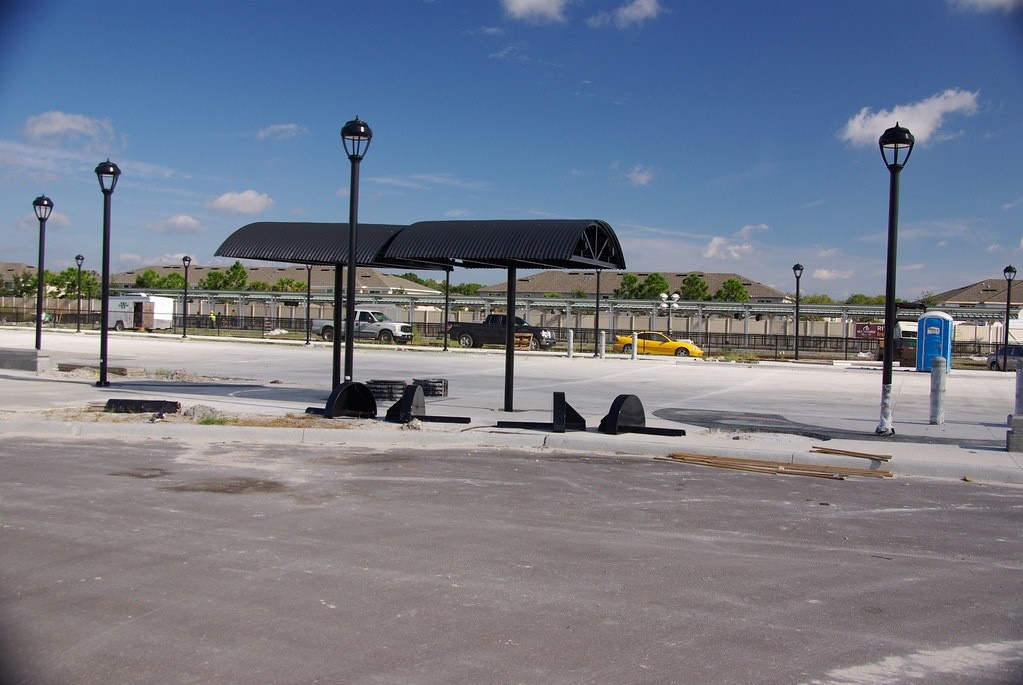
[447,312,557,352]
[310,308,414,346]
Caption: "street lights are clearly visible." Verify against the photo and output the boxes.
[31,191,57,351]
[75,253,85,333]
[337,115,375,381]
[873,120,915,438]
[657,291,680,337]
[1002,264,1017,372]
[791,261,805,360]
[182,255,192,340]
[94,156,125,389]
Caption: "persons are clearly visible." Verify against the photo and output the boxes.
[44,314,53,322]
[41,312,46,320]
[231,309,237,326]
[209,310,216,328]
[218,311,222,328]
[195,312,201,328]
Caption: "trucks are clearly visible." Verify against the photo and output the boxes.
[108,292,175,334]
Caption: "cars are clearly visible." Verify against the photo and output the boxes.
[612,330,703,359]
[987,344,1023,373]
